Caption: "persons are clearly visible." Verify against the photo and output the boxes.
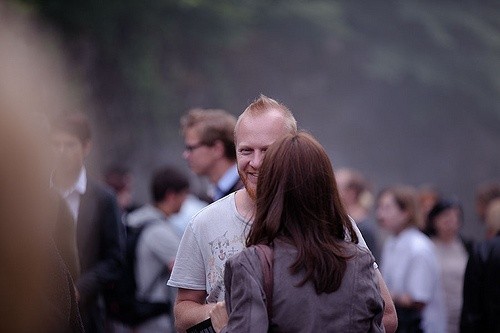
[48,109,208,333]
[167,94,398,333]
[335,166,500,333]
[180,106,244,203]
[209,133,386,333]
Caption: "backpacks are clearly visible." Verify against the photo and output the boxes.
[103,221,144,307]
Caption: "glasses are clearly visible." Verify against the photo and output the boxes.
[185,142,206,150]
